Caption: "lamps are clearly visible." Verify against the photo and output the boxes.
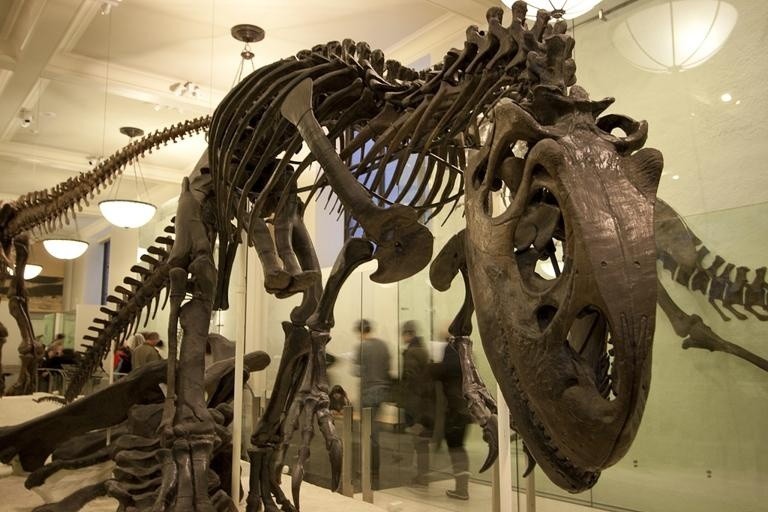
[42,217,89,260]
[612,0,738,75]
[501,0,602,23]
[7,246,43,280]
[98,127,157,229]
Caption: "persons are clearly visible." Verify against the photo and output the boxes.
[406,319,473,499]
[36,331,164,381]
[350,319,390,417]
[400,319,430,381]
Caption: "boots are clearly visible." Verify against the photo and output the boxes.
[445,471,472,499]
